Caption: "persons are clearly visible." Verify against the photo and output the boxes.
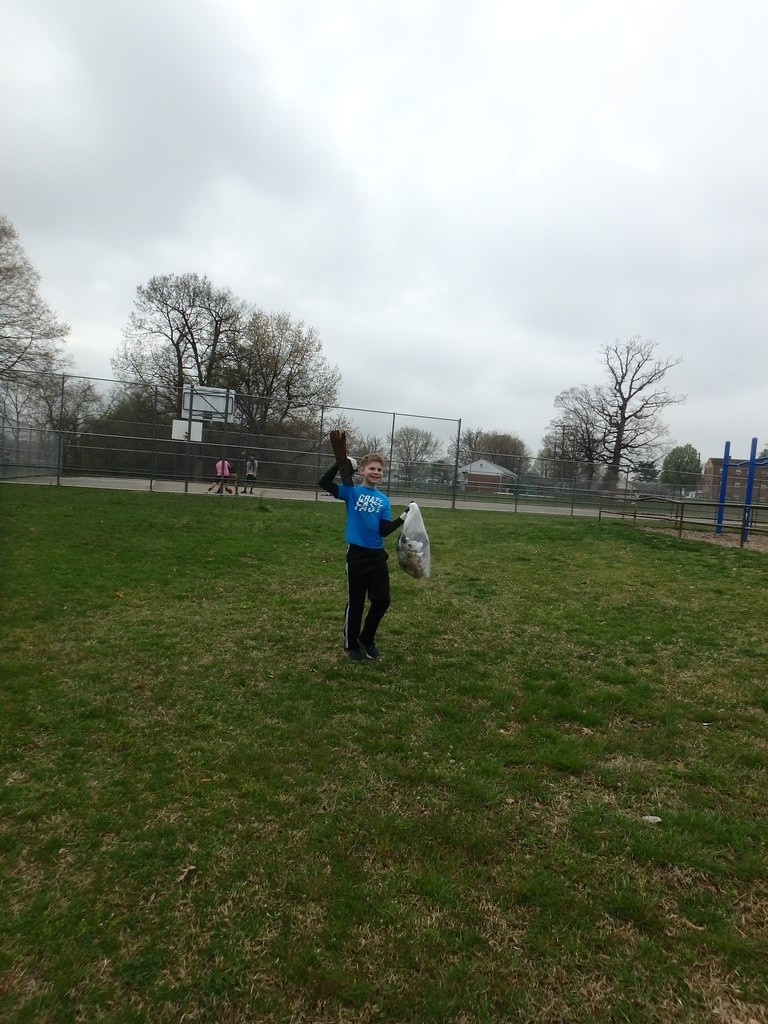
[207,458,233,494]
[319,453,414,661]
[240,454,258,495]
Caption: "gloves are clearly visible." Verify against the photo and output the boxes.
[334,456,358,476]
[400,500,414,521]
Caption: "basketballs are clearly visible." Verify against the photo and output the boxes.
[227,489,232,494]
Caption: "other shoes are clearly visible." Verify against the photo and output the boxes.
[241,489,247,493]
[250,490,253,494]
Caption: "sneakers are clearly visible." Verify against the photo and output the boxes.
[342,642,366,662]
[357,635,381,659]
